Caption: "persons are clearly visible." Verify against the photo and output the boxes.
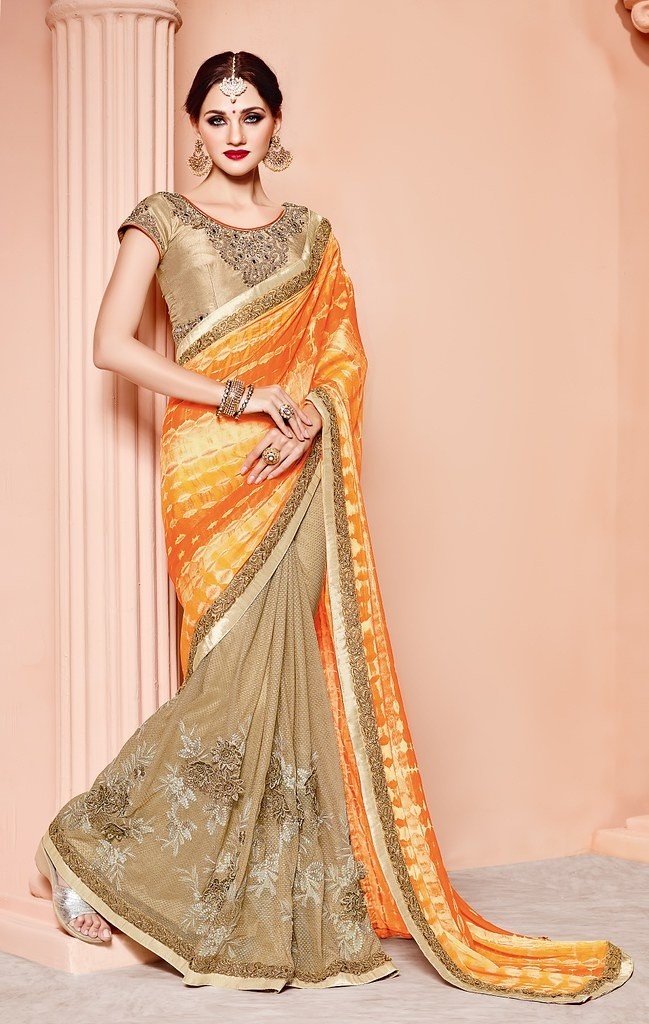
[32,49,368,979]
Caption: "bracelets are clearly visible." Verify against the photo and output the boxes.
[216,379,255,420]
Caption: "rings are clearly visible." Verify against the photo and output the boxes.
[278,400,294,420]
[261,448,280,465]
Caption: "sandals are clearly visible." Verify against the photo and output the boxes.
[33,841,105,943]
